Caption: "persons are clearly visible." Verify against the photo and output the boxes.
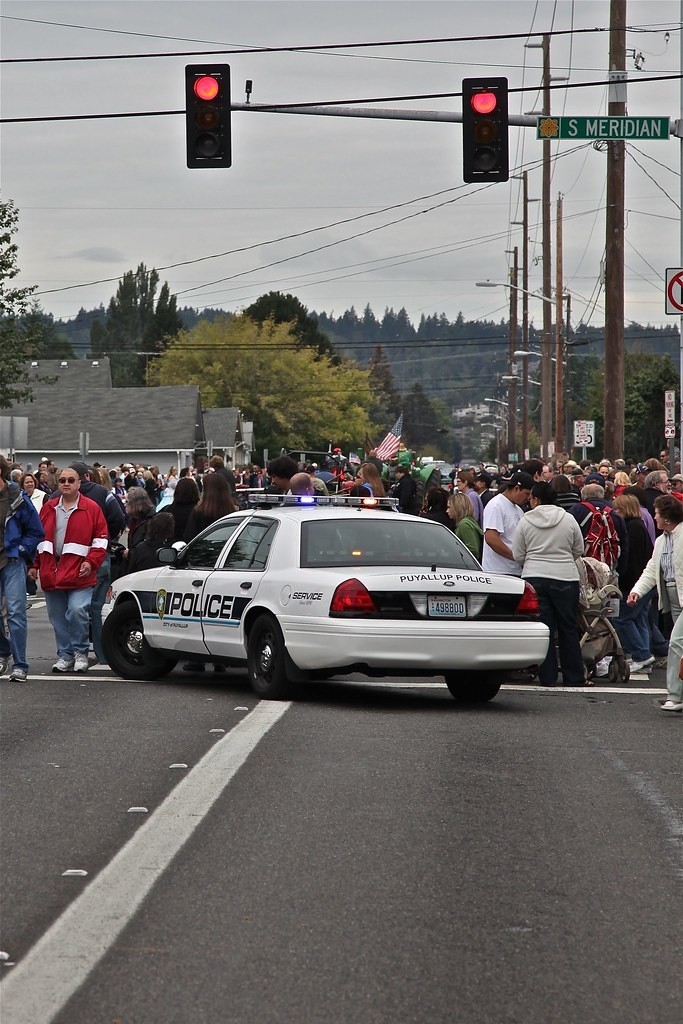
[0,443,683,710]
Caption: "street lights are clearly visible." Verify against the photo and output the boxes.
[478,280,572,461]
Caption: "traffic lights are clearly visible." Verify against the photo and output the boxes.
[462,77,508,183]
[185,64,232,169]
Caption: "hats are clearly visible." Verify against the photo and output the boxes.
[580,460,590,469]
[454,464,459,468]
[9,462,21,470]
[572,468,585,477]
[584,473,605,488]
[635,465,652,474]
[476,472,493,486]
[500,472,534,490]
[668,473,683,484]
[306,466,316,474]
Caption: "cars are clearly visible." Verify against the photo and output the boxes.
[100,494,549,702]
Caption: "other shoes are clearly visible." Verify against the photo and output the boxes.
[661,701,683,710]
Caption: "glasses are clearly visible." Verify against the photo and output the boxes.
[58,477,79,484]
[660,454,668,457]
[356,474,361,480]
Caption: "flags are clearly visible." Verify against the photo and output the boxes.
[375,415,403,461]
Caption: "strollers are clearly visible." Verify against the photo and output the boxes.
[576,555,631,685]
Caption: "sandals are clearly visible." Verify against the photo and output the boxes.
[580,680,594,687]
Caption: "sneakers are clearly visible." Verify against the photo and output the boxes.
[74,652,87,673]
[629,655,655,672]
[8,669,26,682]
[0,654,12,676]
[52,658,74,672]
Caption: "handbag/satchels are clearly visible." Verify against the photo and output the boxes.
[577,502,620,570]
